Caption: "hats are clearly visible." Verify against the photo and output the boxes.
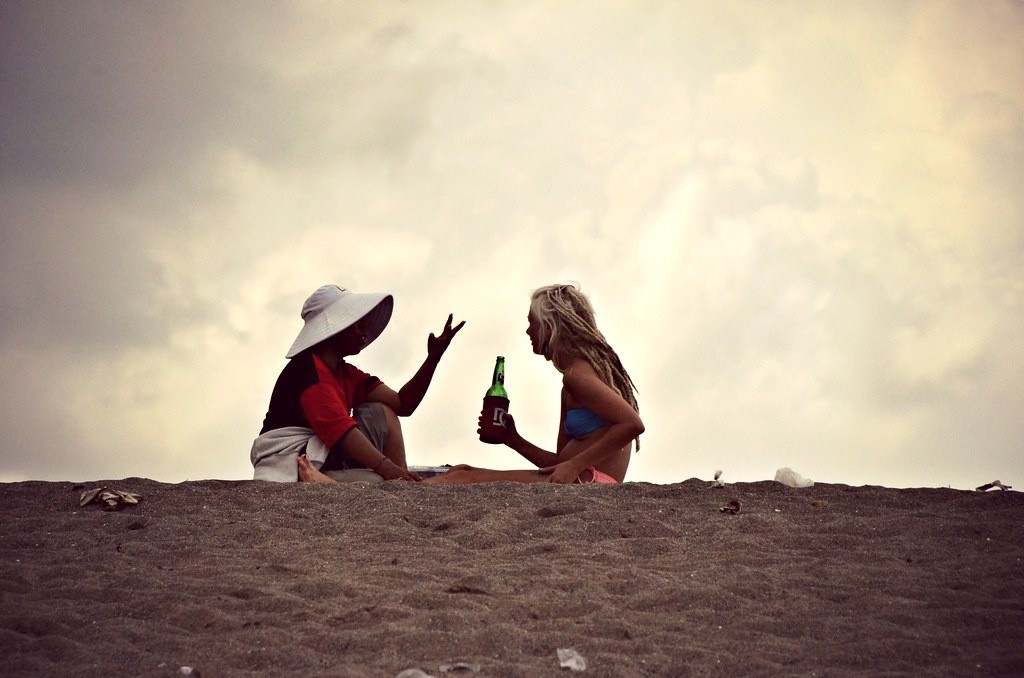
[284,283,393,359]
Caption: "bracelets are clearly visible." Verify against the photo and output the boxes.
[372,456,388,471]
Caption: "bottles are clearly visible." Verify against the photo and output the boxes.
[480,356,508,445]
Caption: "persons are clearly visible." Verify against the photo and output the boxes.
[297,284,645,484]
[250,285,466,481]
